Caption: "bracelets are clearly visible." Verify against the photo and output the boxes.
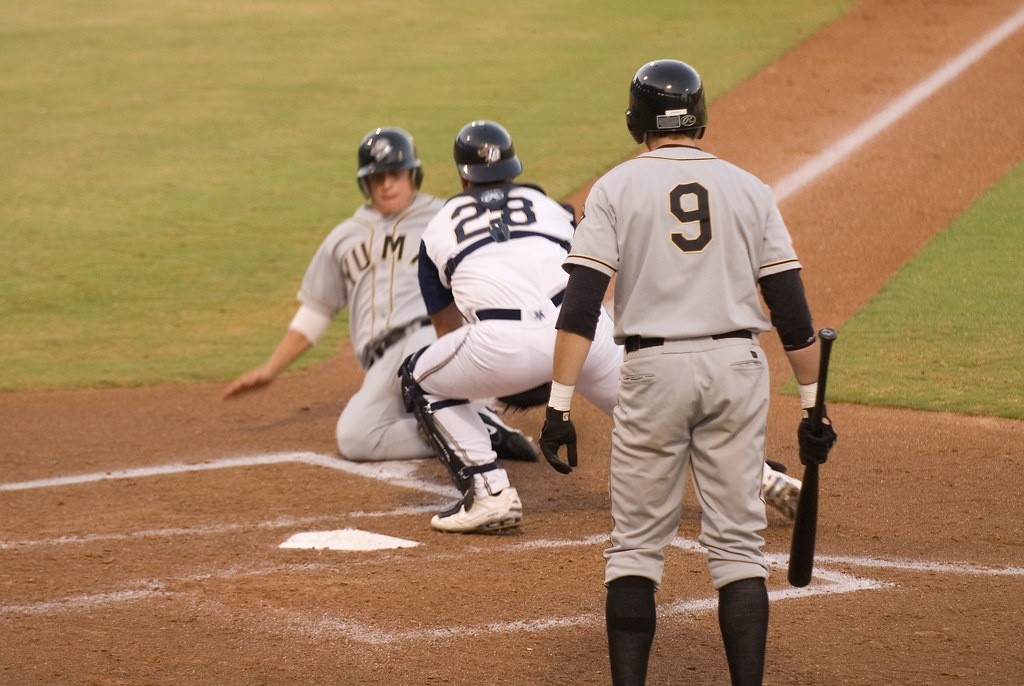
[800,380,818,409]
[547,379,575,411]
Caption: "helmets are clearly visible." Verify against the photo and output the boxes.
[626,59,708,144]
[356,127,424,200]
[454,120,523,182]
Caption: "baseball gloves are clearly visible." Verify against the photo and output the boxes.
[491,376,553,410]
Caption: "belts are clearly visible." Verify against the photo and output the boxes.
[625,330,753,353]
[475,287,565,320]
[366,318,433,368]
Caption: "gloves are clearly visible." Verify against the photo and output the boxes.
[797,405,838,465]
[538,403,578,474]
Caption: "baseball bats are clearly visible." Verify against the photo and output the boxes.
[785,327,838,590]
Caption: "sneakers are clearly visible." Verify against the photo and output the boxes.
[761,460,803,521]
[477,406,538,462]
[430,468,524,535]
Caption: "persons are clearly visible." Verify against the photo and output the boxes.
[222,127,537,461]
[540,57,837,686]
[396,119,808,531]
